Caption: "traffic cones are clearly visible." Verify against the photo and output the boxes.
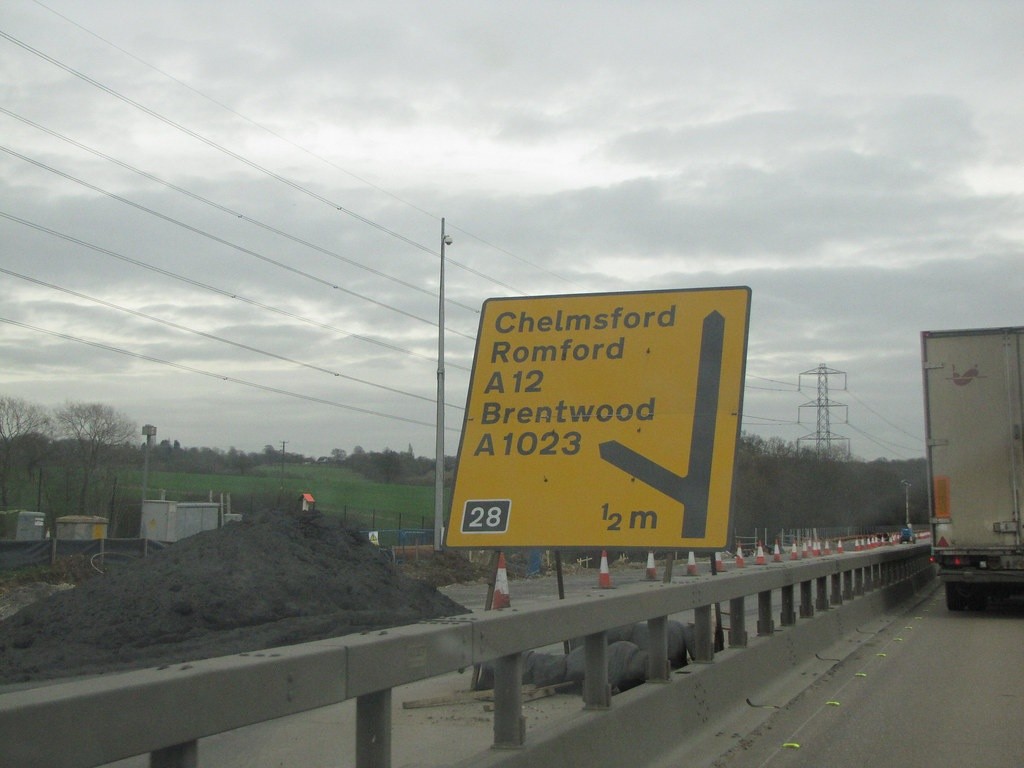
[491,551,511,609]
[736,540,747,568]
[836,538,843,553]
[753,539,767,565]
[789,537,800,561]
[823,540,831,555]
[771,538,783,562]
[801,539,809,558]
[681,550,701,576]
[812,539,818,558]
[855,525,930,551]
[808,537,812,551]
[709,551,727,572]
[639,549,661,582]
[816,540,821,555]
[592,549,617,590]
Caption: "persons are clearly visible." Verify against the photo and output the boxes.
[876,523,909,541]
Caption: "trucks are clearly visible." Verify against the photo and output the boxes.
[919,325,1024,613]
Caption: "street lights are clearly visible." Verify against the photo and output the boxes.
[434,217,453,551]
[902,479,911,527]
